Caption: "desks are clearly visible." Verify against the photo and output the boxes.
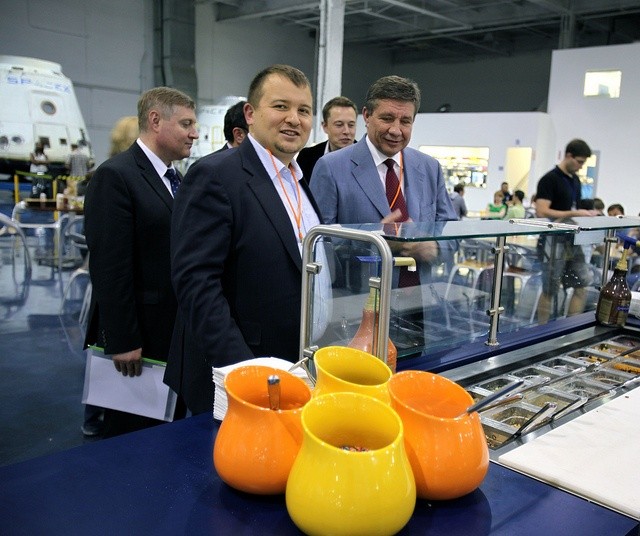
[468,236,544,249]
[1,410,639,536]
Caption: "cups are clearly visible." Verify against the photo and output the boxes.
[389,369,491,501]
[286,392,416,536]
[214,365,311,497]
[309,346,391,407]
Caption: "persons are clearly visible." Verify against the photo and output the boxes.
[309,75,462,293]
[527,196,537,218]
[607,204,637,256]
[484,191,507,220]
[78,171,96,197]
[503,191,526,220]
[578,199,596,210]
[592,196,604,216]
[534,139,602,325]
[189,100,259,169]
[296,96,361,187]
[80,116,143,436]
[29,141,50,198]
[82,86,199,437]
[449,184,467,221]
[66,143,95,177]
[501,182,511,204]
[161,64,336,417]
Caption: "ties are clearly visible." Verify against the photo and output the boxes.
[382,159,408,222]
[164,170,181,197]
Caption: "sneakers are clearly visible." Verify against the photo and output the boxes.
[81,419,101,436]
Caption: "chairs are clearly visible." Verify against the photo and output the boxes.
[445,244,496,293]
[503,249,543,316]
